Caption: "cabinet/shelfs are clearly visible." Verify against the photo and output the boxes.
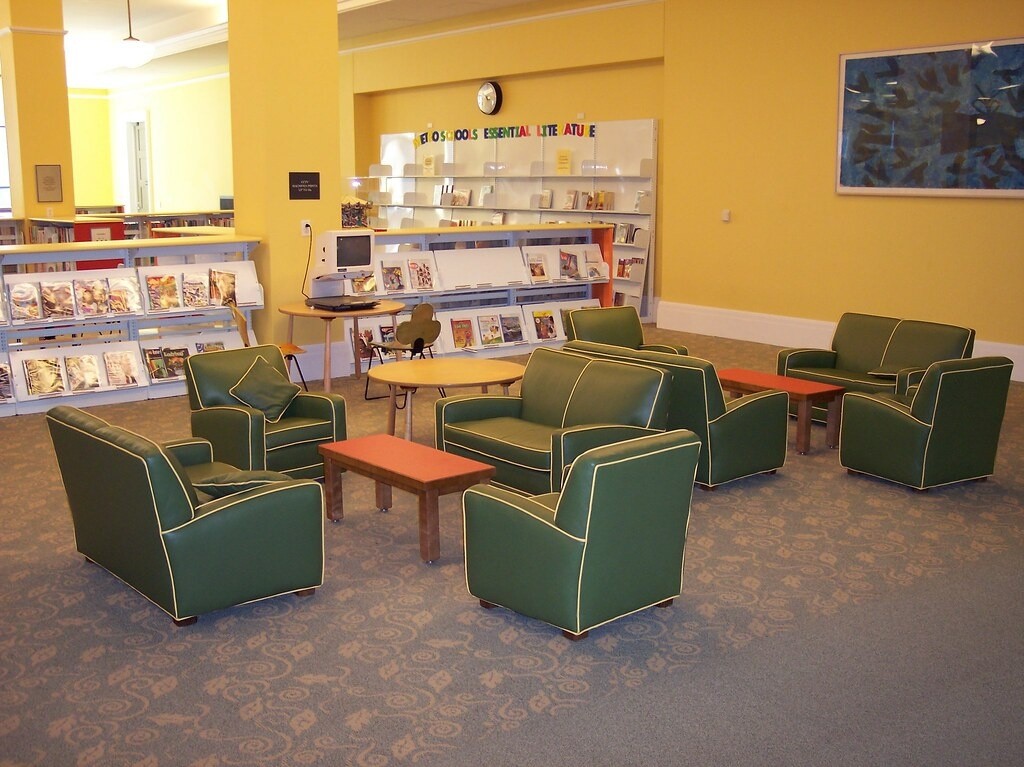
[345,118,657,323]
[0,235,264,418]
[344,223,615,374]
[0,204,235,274]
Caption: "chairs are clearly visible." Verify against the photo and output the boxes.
[227,303,309,393]
[364,302,447,409]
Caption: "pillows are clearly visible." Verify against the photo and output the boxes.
[191,470,293,498]
[867,366,906,379]
[228,355,301,424]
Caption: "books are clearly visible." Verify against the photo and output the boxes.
[210,217,234,227]
[147,276,180,310]
[452,185,648,306]
[22,358,65,395]
[450,250,603,348]
[347,258,433,359]
[164,217,198,227]
[162,348,190,377]
[104,350,141,386]
[195,341,225,353]
[64,355,99,391]
[133,255,158,266]
[208,267,237,307]
[29,225,74,244]
[143,346,169,381]
[36,261,75,272]
[182,272,210,308]
[0,363,14,398]
[124,224,139,230]
[0,264,145,326]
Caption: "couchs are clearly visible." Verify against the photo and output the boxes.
[44,405,325,627]
[461,429,703,642]
[561,340,790,492]
[183,344,348,484]
[565,305,689,357]
[776,313,976,424]
[838,357,1014,494]
[433,346,672,499]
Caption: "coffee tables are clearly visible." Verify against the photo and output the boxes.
[319,434,496,566]
[717,367,846,455]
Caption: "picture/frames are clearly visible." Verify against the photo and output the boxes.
[835,36,1024,200]
[35,165,63,202]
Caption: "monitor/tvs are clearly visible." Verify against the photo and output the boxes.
[314,230,374,281]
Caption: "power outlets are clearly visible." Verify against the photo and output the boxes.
[301,220,311,236]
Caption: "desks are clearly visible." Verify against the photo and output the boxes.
[367,357,526,443]
[279,301,406,393]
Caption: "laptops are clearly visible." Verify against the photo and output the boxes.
[304,296,381,311]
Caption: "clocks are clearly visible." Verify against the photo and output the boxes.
[477,82,502,116]
